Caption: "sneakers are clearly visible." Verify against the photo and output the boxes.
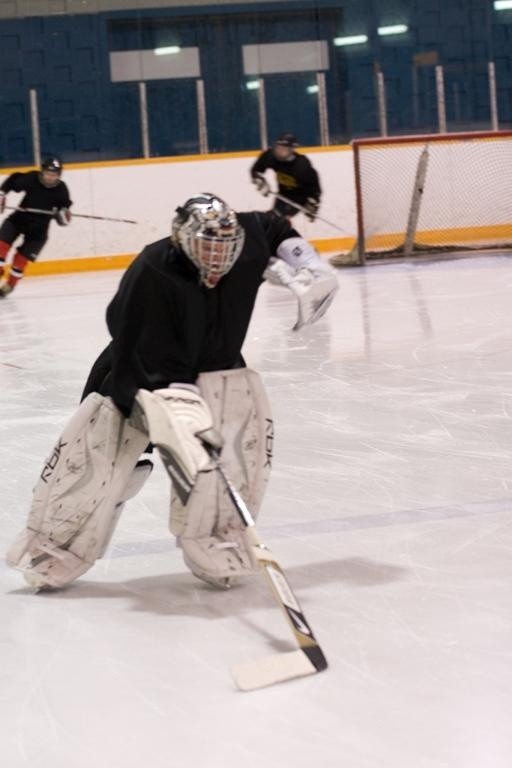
[0,282,14,297]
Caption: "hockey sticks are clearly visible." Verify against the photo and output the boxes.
[268,189,358,235]
[4,206,155,227]
[202,441,329,690]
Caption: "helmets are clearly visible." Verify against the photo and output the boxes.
[38,157,63,187]
[272,132,299,160]
[169,192,247,289]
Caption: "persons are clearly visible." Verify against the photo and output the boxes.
[6,190,341,589]
[250,128,321,224]
[0,155,73,298]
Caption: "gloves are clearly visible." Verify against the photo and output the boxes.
[52,206,72,226]
[254,176,269,197]
[0,190,6,214]
[304,196,319,222]
